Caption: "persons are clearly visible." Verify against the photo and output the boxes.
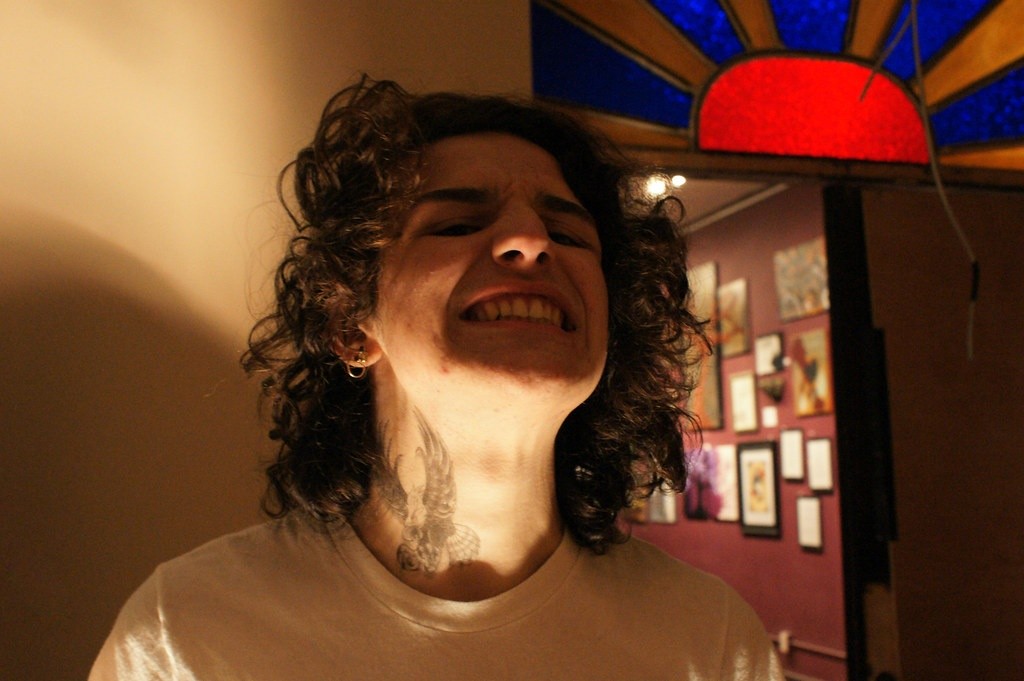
[89,74,784,681]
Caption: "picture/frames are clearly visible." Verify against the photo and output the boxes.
[628,239,834,555]
